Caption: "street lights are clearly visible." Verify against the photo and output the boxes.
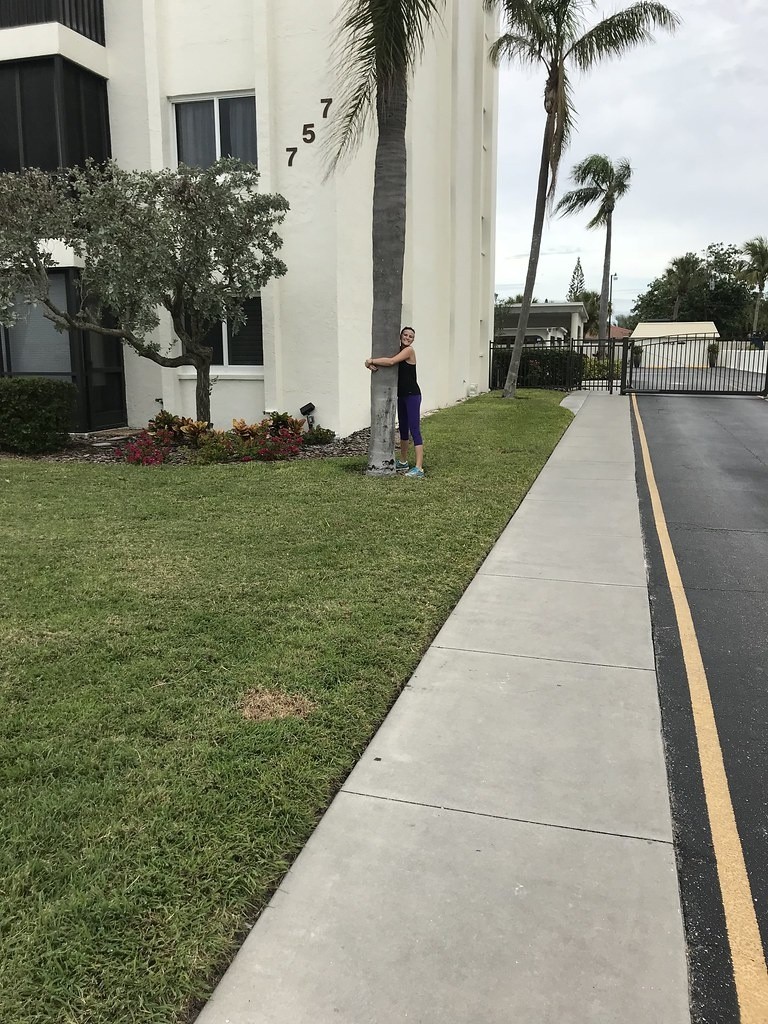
[608,273,618,338]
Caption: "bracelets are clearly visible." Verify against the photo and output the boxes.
[372,359,373,363]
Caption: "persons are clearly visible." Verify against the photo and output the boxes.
[365,327,425,477]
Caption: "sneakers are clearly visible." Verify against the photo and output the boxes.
[405,467,424,477]
[396,460,409,470]
